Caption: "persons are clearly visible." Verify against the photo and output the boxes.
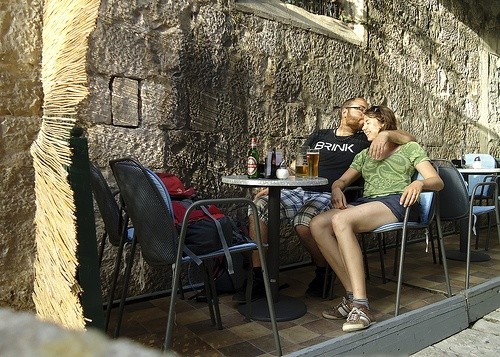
[232,98,417,302]
[309,105,445,331]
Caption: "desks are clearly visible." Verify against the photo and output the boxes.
[221,172,329,322]
[446,166,500,262]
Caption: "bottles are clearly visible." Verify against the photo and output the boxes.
[271,148,277,179]
[248,134,259,179]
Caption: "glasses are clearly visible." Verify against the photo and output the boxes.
[345,106,367,112]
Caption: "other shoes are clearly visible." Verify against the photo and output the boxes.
[322,295,355,320]
[305,265,336,298]
[231,275,265,303]
[342,301,375,332]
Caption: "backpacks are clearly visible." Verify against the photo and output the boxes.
[154,172,235,257]
[205,230,254,296]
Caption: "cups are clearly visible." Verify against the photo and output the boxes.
[276,169,289,180]
[295,145,310,179]
[306,149,320,178]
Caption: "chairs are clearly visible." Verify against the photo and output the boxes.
[106,158,282,356]
[318,161,452,318]
[86,160,223,338]
[426,154,500,264]
